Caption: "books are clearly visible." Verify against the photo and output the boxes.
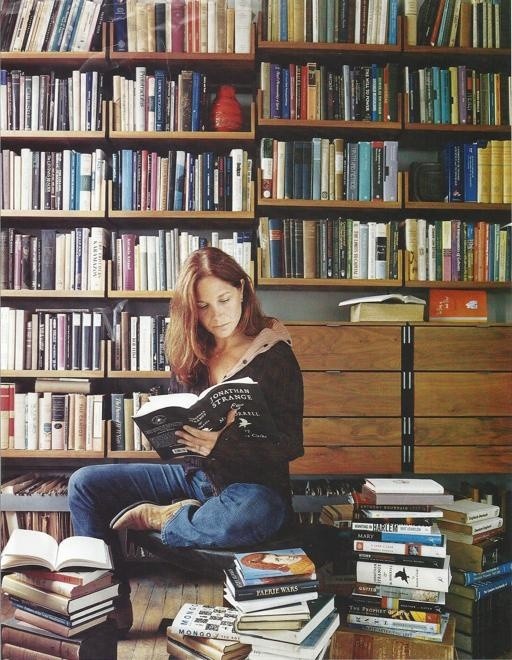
[131,377,280,463]
[166,547,340,660]
[289,478,512,660]
[0,307,171,452]
[0,1,512,322]
[0,473,157,660]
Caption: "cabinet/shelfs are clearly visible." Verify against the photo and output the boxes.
[0,0,510,481]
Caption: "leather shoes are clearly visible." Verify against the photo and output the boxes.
[108,496,202,532]
[108,603,132,640]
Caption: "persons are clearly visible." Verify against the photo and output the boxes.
[69,247,304,632]
